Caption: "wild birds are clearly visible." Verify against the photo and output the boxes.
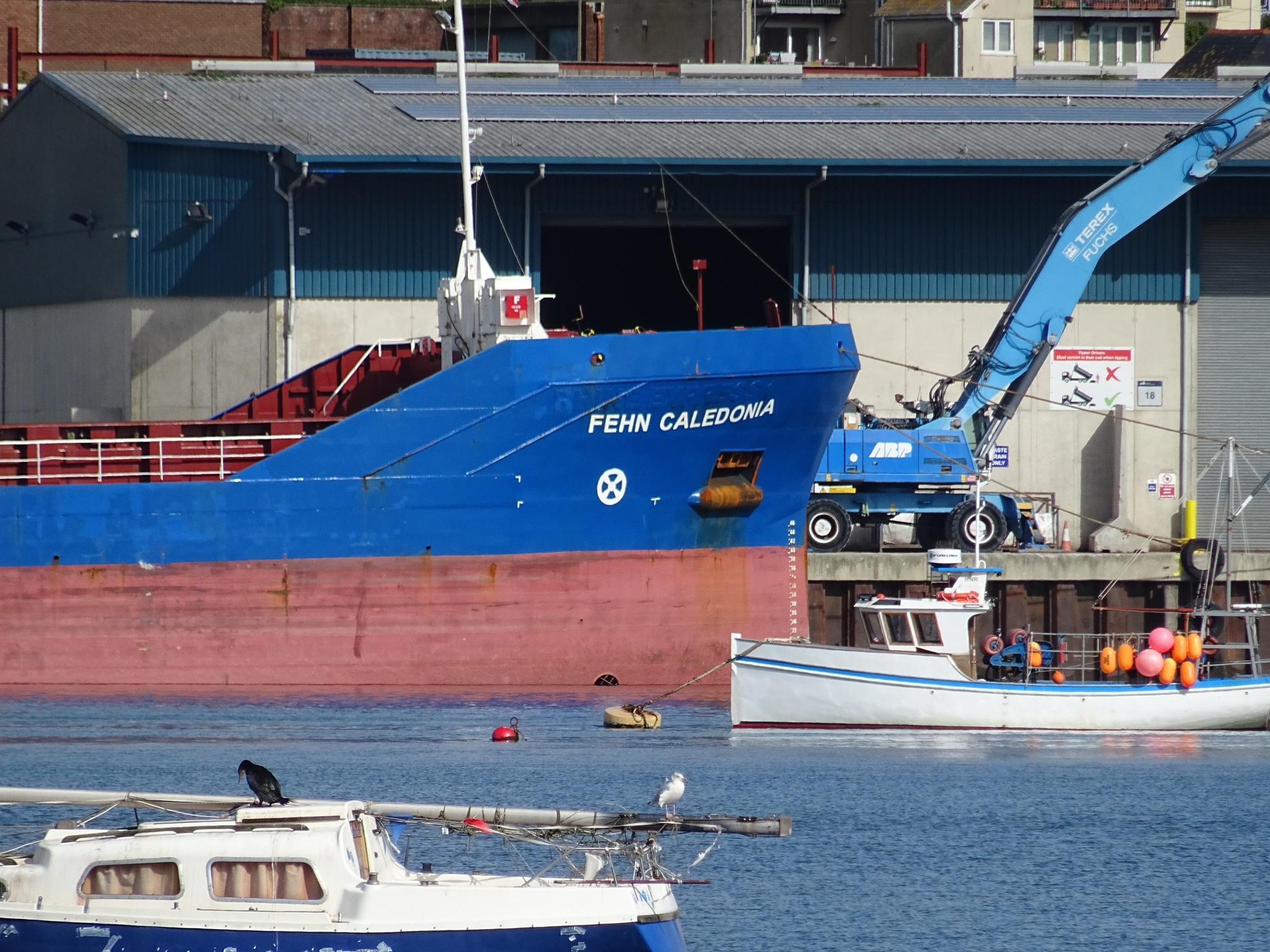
[237,759,295,807]
[647,772,688,814]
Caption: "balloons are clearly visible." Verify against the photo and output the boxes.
[1148,627,1174,652]
[1135,649,1164,677]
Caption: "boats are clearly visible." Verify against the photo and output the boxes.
[602,437,1270,734]
[0,787,791,952]
[1,3,866,701]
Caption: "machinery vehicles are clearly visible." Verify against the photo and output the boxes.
[807,68,1270,556]
